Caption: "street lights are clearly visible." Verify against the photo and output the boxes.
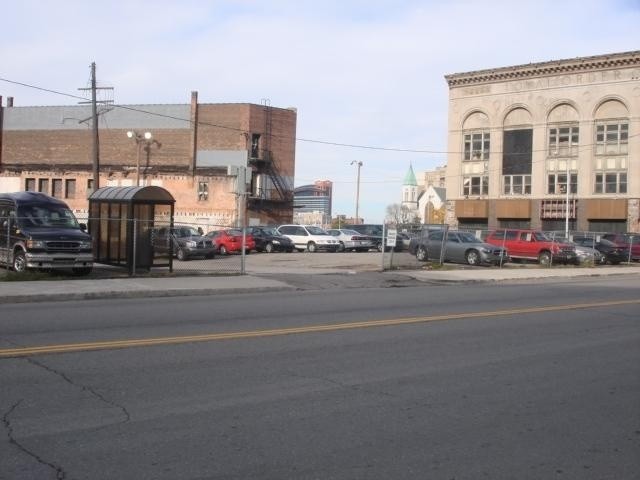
[350,159,363,224]
[126,130,152,186]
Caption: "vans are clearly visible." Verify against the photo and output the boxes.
[0,191,95,275]
